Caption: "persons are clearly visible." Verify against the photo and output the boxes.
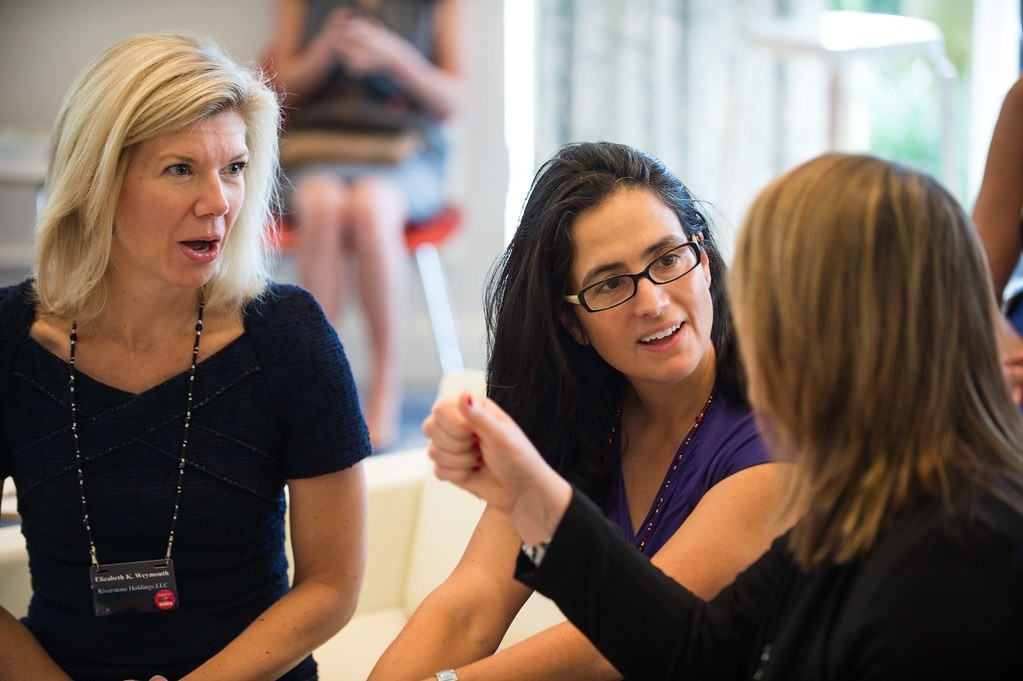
[971,78,1022,416]
[257,2,466,460]
[2,37,374,677]
[420,152,1020,678]
[366,138,806,681]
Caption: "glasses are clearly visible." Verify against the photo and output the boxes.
[562,232,702,313]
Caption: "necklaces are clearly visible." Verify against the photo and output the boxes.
[596,380,721,550]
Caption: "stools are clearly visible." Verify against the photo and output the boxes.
[261,208,473,416]
